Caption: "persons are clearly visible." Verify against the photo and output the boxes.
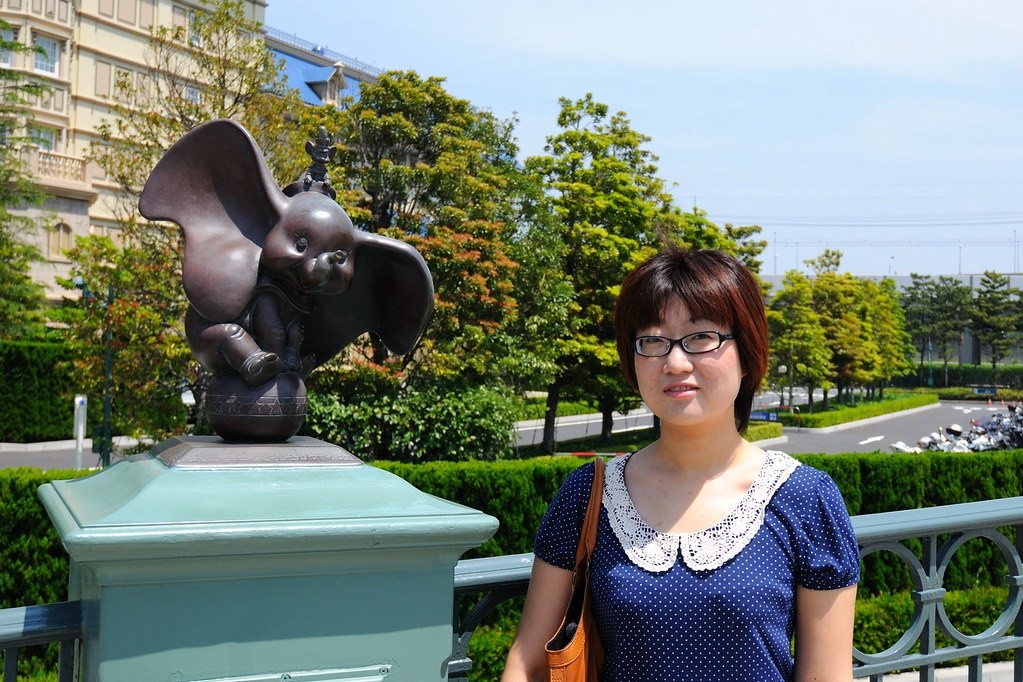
[501,250,860,682]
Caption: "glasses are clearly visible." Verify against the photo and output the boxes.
[634,330,734,357]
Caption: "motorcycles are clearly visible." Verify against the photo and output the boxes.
[891,404,1023,453]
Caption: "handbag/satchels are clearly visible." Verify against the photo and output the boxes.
[545,457,607,682]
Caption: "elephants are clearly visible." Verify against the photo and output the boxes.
[138,119,434,390]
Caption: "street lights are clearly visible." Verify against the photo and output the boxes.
[772,231,778,273]
[888,256,894,275]
[777,363,788,406]
[1013,228,1016,272]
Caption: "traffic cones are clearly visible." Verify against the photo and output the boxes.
[1001,400,1005,407]
[988,398,993,406]
